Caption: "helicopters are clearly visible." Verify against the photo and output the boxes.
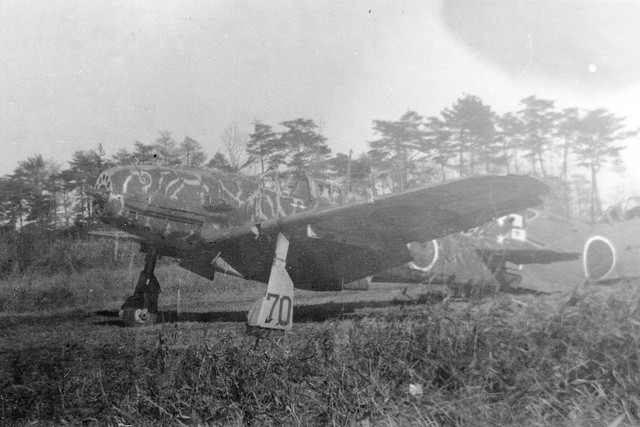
[83,166,579,340]
